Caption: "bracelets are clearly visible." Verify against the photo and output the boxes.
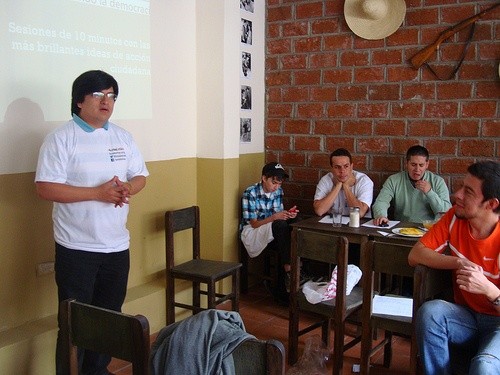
[488,295,500,306]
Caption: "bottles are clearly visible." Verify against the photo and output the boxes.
[349,206,360,228]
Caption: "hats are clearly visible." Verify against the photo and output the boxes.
[262,161,290,179]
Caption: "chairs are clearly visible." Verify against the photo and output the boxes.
[60,298,151,375]
[288,228,380,375]
[165,206,243,326]
[364,241,455,375]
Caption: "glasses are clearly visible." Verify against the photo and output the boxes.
[89,91,117,101]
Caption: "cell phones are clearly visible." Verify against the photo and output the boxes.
[289,206,297,212]
[379,222,389,227]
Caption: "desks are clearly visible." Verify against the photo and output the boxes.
[289,215,426,245]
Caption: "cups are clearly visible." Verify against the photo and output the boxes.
[332,207,343,228]
[423,219,436,229]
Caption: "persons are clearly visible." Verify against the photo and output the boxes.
[313,148,374,220]
[372,145,453,226]
[241,162,306,293]
[408,161,500,375]
[35,70,148,375]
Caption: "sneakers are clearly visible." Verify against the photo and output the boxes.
[285,272,324,293]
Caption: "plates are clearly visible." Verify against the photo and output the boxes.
[392,227,425,237]
